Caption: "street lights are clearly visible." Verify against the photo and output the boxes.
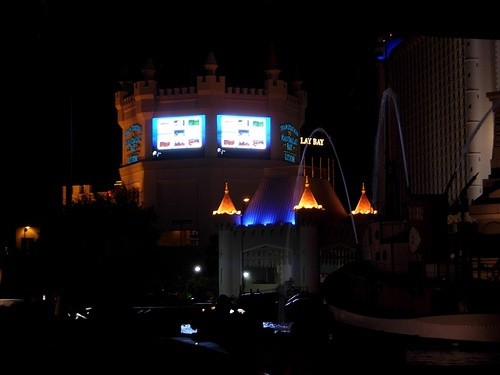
[235,195,252,295]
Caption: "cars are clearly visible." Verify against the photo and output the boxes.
[0,288,372,375]
